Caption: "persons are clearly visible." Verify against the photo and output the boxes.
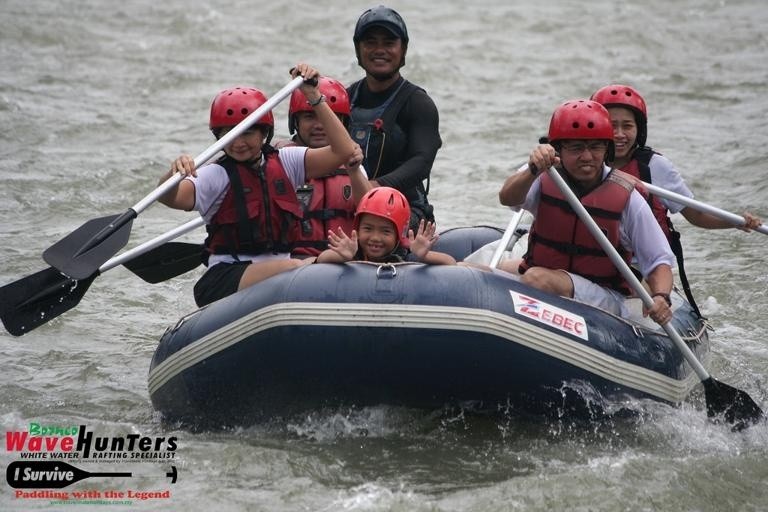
[345,5,442,239]
[268,74,374,262]
[156,63,355,310]
[589,85,763,299]
[455,98,675,327]
[312,185,458,266]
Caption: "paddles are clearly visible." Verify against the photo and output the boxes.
[531,151,762,433]
[121,242,208,284]
[43,65,318,280]
[0,216,206,336]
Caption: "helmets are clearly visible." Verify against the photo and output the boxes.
[591,85,646,148]
[210,9,410,243]
[548,100,614,163]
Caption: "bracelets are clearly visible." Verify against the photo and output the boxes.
[307,94,326,107]
[650,292,672,308]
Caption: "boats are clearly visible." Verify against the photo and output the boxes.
[146,226,710,425]
